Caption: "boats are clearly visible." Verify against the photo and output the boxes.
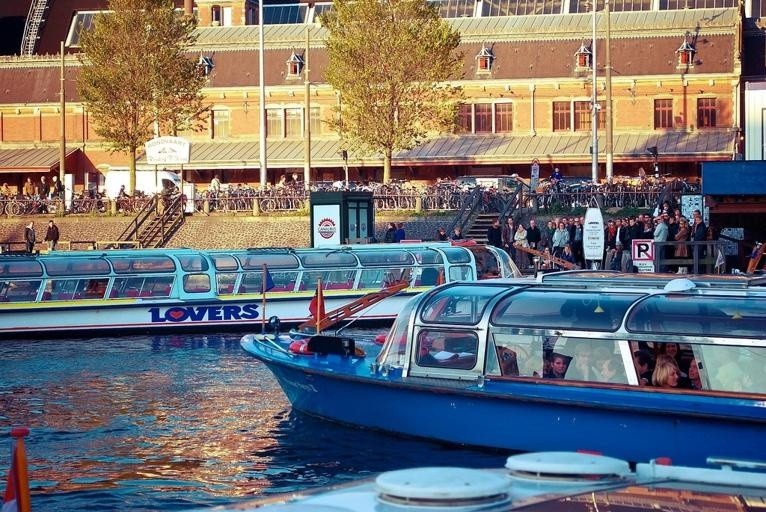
[2,238,530,344]
[238,270,764,475]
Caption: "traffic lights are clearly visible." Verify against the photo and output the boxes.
[336,150,346,159]
[645,146,658,156]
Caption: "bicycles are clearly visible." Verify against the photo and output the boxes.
[0,179,704,218]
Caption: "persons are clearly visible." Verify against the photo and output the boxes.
[549,167,562,186]
[211,175,220,194]
[38,175,50,199]
[119,185,131,199]
[23,221,36,253]
[487,201,708,274]
[487,325,706,390]
[43,220,59,251]
[435,227,449,241]
[452,227,464,240]
[385,223,397,243]
[393,223,405,243]
[290,173,298,187]
[24,177,36,200]
[52,176,60,197]
